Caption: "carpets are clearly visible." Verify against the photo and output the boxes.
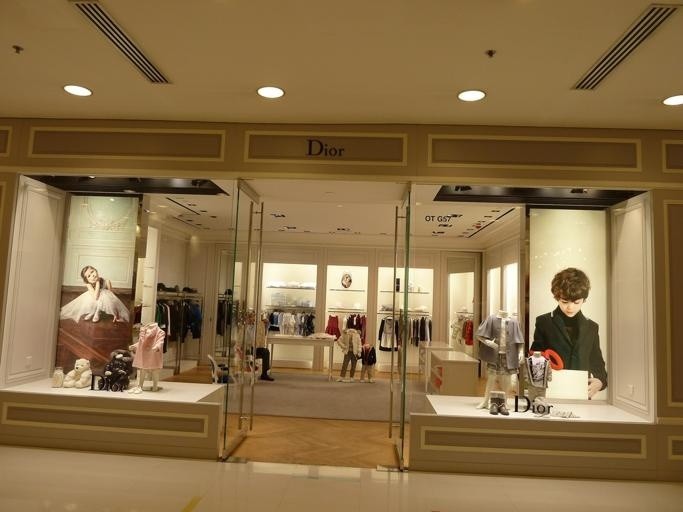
[196,379,437,422]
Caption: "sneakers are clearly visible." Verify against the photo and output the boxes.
[92,315,99,323]
[84,314,93,321]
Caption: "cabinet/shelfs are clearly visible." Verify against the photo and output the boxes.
[429,350,480,396]
[262,284,431,317]
[417,341,455,385]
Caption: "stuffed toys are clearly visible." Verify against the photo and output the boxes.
[98,348,133,393]
[62,358,94,389]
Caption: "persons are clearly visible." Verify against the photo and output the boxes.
[56,265,132,324]
[530,267,607,400]
[474,308,525,410]
[126,320,166,395]
[335,329,363,383]
[519,350,553,403]
[356,343,376,383]
[245,311,275,382]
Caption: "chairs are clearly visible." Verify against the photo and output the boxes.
[208,354,237,384]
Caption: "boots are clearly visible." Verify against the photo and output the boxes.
[489,391,498,415]
[498,391,509,415]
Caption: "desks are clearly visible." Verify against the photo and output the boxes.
[265,334,338,382]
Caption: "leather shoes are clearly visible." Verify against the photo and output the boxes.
[261,374,274,381]
